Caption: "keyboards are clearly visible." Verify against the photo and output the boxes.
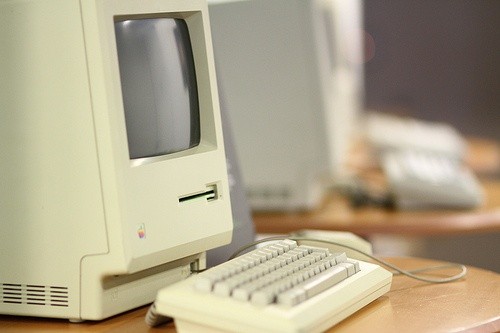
[151,238,394,333]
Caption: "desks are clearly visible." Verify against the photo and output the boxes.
[248,168,500,243]
[0,253,500,333]
[364,117,500,172]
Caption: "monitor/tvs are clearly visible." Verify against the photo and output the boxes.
[0,0,234,322]
